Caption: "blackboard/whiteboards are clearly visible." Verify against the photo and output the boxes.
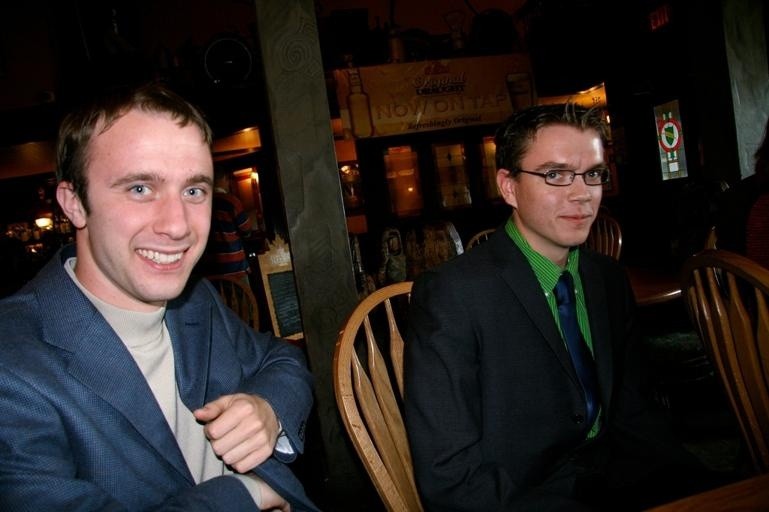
[262,270,304,341]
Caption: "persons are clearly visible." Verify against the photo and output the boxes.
[0,83,320,512]
[402,103,693,511]
[205,165,254,322]
[714,112,769,397]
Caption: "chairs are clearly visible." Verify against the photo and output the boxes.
[333,281,422,512]
[464,214,683,311]
[647,249,769,512]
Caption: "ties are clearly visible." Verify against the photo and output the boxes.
[553,271,600,436]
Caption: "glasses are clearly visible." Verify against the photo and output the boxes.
[519,166,610,186]
